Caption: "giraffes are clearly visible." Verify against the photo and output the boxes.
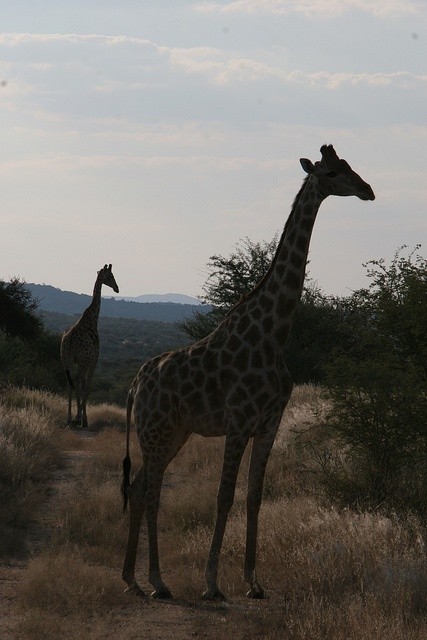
[60,264,119,428]
[120,146,376,602]
